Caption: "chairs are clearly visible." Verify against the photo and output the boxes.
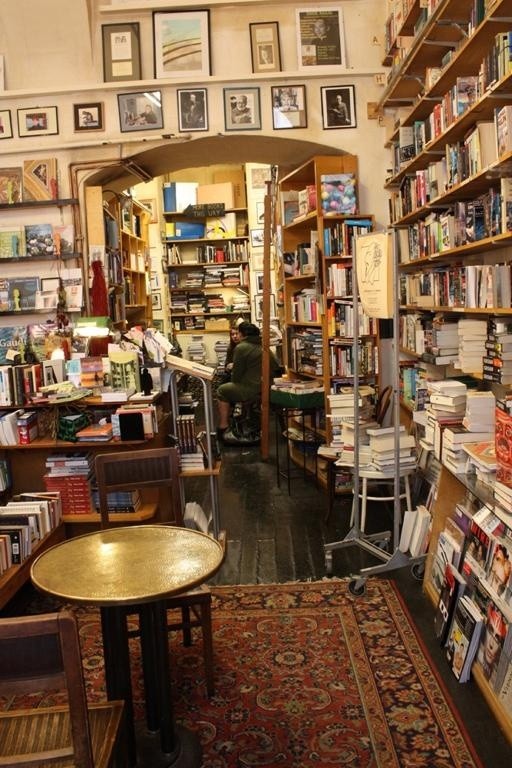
[0,610,125,768]
[92,445,217,699]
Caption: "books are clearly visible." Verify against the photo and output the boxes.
[272,172,418,473]
[1,158,250,575]
[378,1,511,704]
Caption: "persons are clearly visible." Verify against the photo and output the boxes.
[216,321,282,442]
[28,104,157,130]
[274,90,350,126]
[182,94,252,128]
[259,17,340,64]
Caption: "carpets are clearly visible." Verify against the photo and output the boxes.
[0,576,483,768]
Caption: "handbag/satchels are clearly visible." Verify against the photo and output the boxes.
[222,417,262,445]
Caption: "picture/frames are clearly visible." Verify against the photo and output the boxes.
[100,22,142,83]
[177,88,208,133]
[117,90,164,132]
[249,22,282,72]
[321,84,358,132]
[222,87,262,131]
[254,294,276,321]
[253,252,275,272]
[18,105,59,138]
[255,272,272,293]
[256,202,264,224]
[271,85,308,130]
[72,101,104,133]
[1,111,12,139]
[151,8,211,80]
[295,6,345,71]
[250,229,273,247]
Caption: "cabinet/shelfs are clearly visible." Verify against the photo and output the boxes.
[278,155,369,540]
[1,151,253,607]
[370,2,512,738]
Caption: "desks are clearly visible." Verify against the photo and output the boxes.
[29,524,225,768]
